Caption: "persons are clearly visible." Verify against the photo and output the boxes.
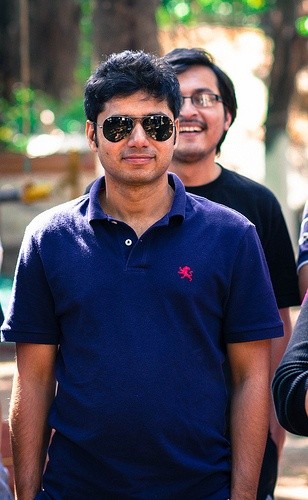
[0,49,283,500]
[272,290,308,438]
[83,47,299,500]
[296,199,308,306]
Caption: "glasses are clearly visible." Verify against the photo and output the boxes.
[95,116,176,144]
[181,93,222,108]
